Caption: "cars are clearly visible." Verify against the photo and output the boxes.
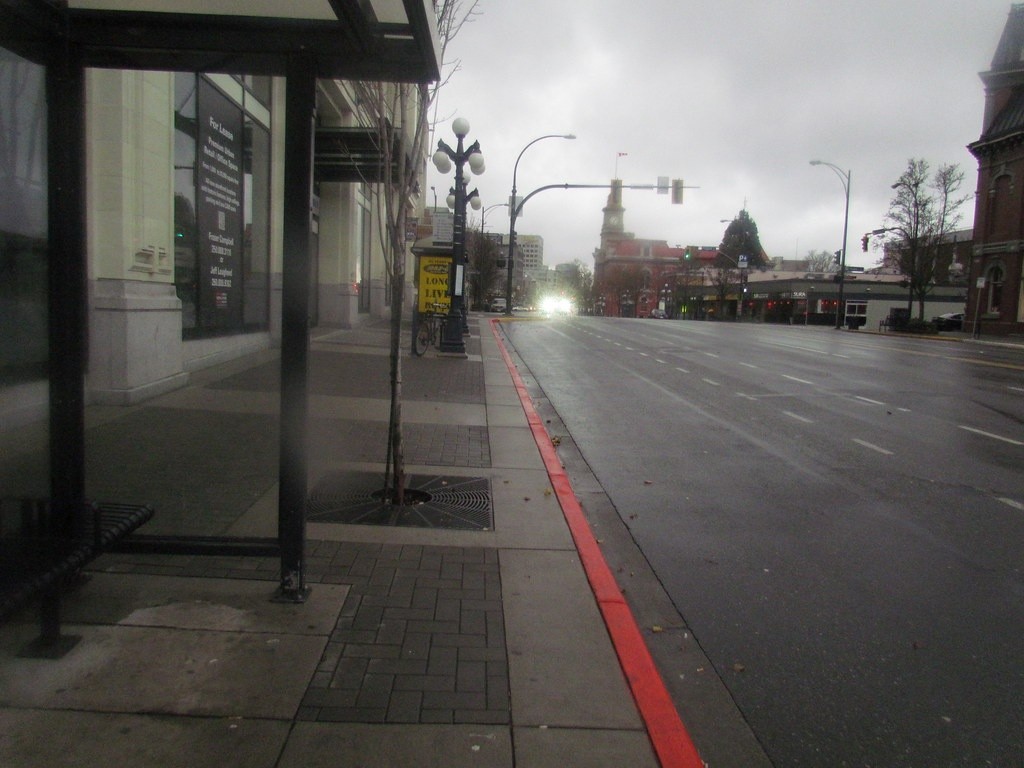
[931,311,963,333]
[489,298,507,313]
[511,303,544,313]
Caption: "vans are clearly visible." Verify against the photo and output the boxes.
[622,303,666,319]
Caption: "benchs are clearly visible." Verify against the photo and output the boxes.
[0,502,156,650]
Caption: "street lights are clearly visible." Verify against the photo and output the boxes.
[809,159,851,330]
[501,132,576,318]
[446,170,484,339]
[431,115,486,359]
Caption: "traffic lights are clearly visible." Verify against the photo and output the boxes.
[861,239,865,250]
[833,251,838,264]
[684,248,689,261]
